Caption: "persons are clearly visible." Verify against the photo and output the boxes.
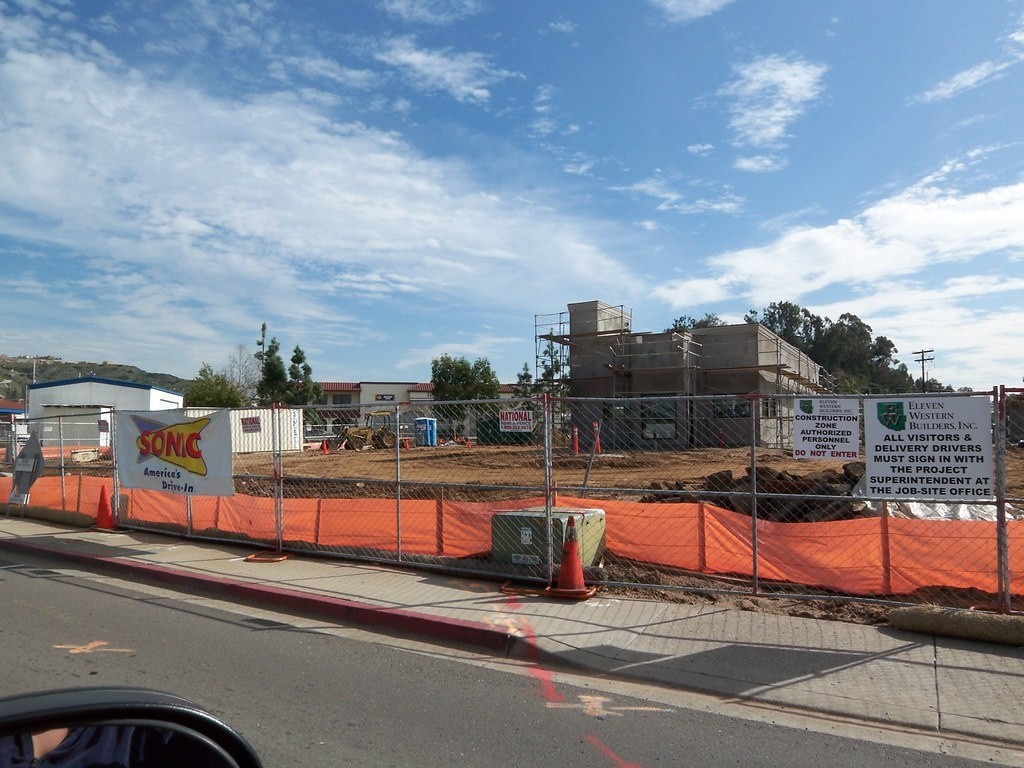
[0,723,225,768]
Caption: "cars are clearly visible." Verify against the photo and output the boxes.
[303,429,334,442]
[0,687,263,768]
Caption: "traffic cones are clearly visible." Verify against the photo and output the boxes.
[554,516,590,594]
[468,440,471,448]
[405,440,410,450]
[90,485,115,531]
[320,440,330,455]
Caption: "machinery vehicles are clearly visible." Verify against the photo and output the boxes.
[340,411,396,449]
